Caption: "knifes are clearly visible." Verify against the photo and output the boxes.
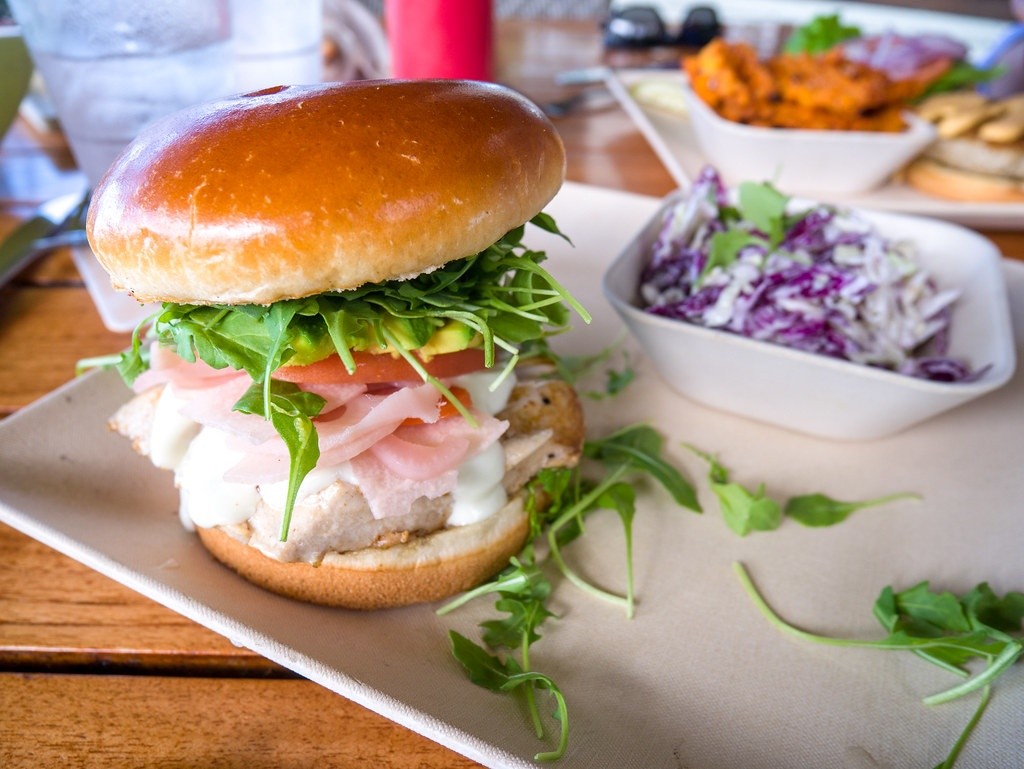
[540,86,616,121]
[0,178,91,288]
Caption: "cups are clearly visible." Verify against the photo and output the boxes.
[382,0,494,88]
[6,0,326,221]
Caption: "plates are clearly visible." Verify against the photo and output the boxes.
[602,65,1024,230]
[0,180,1024,769]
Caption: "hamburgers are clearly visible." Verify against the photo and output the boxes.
[85,79,584,613]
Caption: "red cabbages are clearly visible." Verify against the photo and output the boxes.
[633,170,986,384]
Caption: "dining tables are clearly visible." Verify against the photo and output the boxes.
[0,14,1024,769]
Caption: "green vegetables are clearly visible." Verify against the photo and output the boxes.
[426,415,1024,767]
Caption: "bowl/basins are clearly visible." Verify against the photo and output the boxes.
[603,184,1017,445]
[676,76,937,206]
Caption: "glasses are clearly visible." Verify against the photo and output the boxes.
[598,5,725,50]
